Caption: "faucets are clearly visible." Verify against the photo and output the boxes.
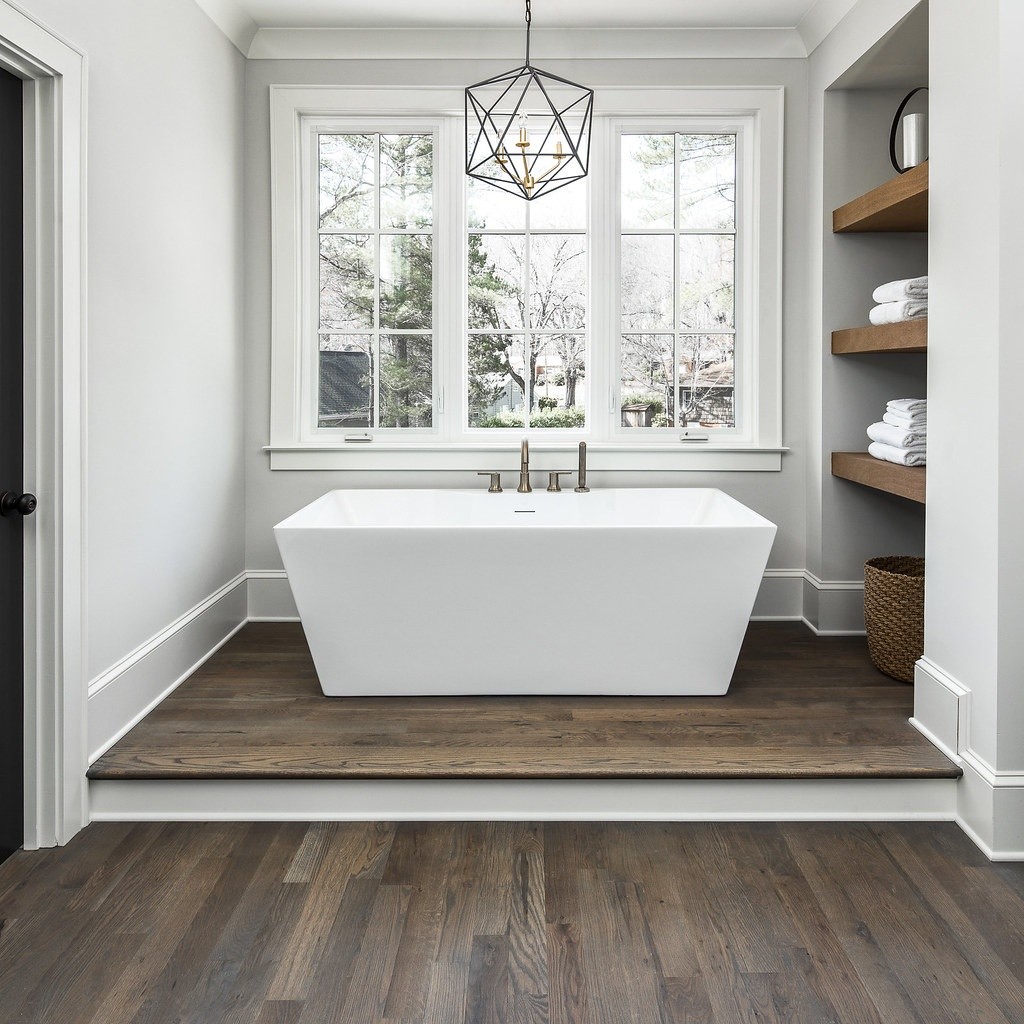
[517,440,533,493]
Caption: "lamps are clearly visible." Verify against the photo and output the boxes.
[464,0,593,201]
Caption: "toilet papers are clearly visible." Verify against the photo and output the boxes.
[902,113,928,169]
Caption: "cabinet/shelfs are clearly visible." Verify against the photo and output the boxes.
[832,157,929,505]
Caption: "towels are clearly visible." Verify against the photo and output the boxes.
[866,398,927,466]
[868,274,927,326]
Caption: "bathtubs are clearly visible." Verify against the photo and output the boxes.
[273,487,779,696]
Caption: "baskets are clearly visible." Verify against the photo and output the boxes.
[864,555,924,683]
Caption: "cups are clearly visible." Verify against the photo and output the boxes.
[903,112,927,169]
